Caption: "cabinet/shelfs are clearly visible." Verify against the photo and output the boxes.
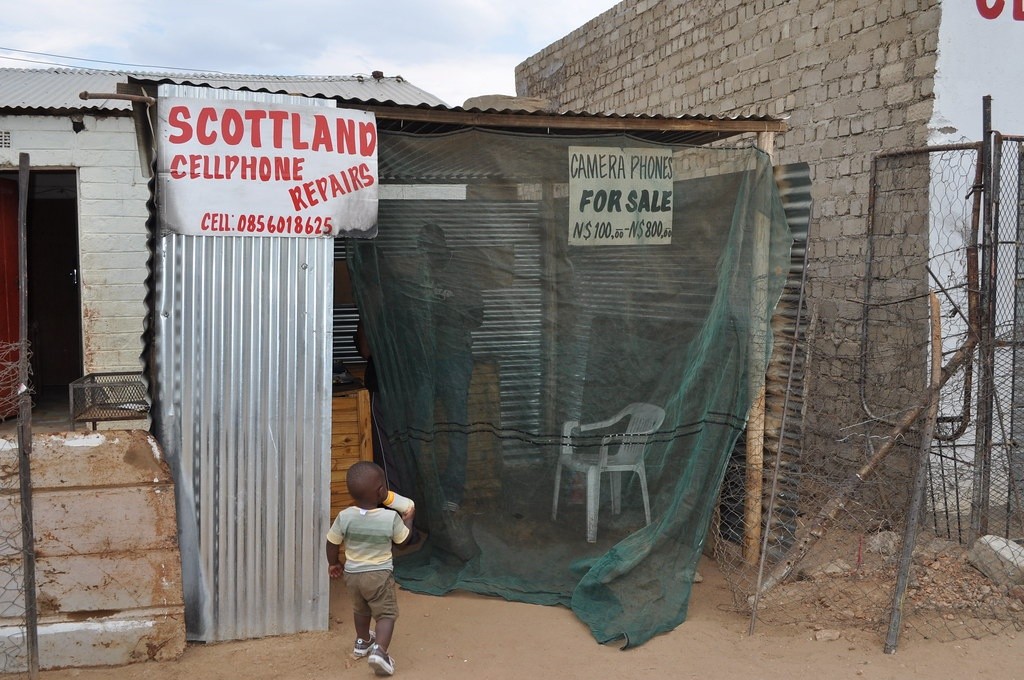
[331,358,503,569]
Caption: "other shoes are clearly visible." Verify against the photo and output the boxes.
[441,500,460,518]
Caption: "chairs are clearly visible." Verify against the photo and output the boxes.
[552,402,666,542]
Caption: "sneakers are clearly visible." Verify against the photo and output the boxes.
[353,630,376,657]
[368,644,395,675]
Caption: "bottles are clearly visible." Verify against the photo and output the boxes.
[382,491,415,514]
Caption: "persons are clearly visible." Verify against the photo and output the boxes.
[324,460,415,676]
[354,223,486,545]
